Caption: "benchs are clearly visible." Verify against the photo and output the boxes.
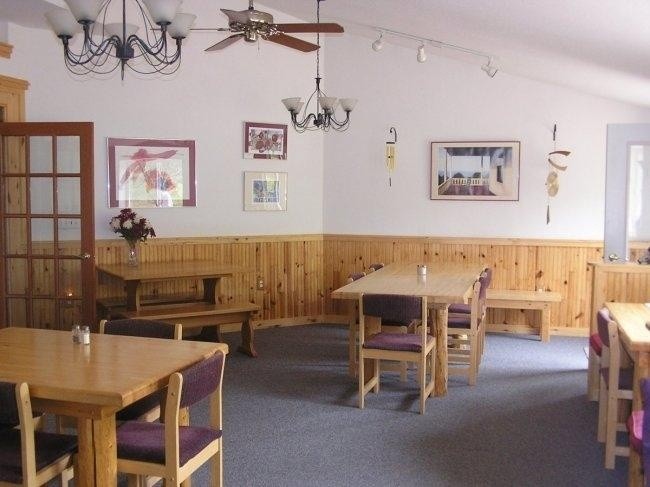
[487,288,561,343]
[93,294,196,316]
[111,302,261,359]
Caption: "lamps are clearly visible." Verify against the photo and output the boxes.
[372,27,498,79]
[283,1,357,134]
[43,0,197,82]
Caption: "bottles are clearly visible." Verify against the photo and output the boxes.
[421,265,426,274]
[79,325,90,344]
[71,324,80,342]
[416,264,421,273]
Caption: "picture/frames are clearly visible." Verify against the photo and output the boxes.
[431,140,520,201]
[107,137,196,207]
[245,121,287,159]
[244,170,288,211]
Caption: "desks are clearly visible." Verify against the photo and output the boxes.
[604,302,649,487]
[332,260,485,398]
[96,261,233,345]
[0,326,229,487]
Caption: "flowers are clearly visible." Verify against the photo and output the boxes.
[109,208,155,248]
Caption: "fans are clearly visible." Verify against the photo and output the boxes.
[149,0,344,53]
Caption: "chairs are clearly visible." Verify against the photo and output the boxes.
[368,263,384,273]
[428,278,486,384]
[595,306,633,470]
[628,411,644,485]
[448,266,493,349]
[116,350,225,486]
[358,290,436,413]
[54,319,183,487]
[346,272,408,382]
[590,332,600,402]
[0,381,77,487]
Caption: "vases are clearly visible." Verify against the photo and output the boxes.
[128,248,138,266]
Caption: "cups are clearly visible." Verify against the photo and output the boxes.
[537,283,545,294]
